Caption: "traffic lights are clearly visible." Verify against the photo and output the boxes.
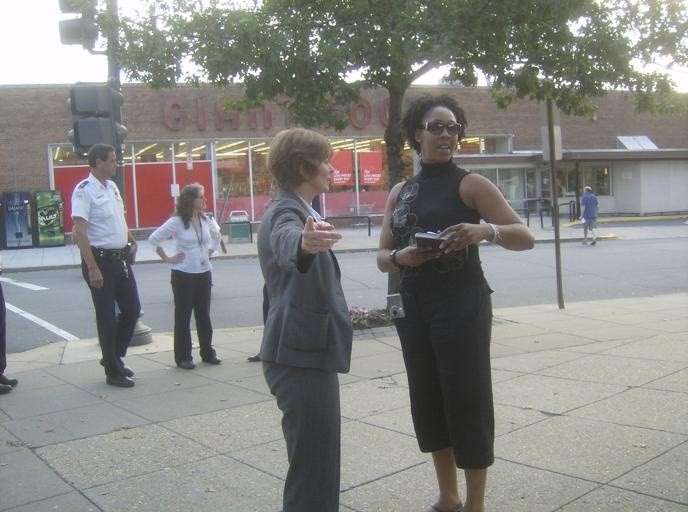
[58,0,99,46]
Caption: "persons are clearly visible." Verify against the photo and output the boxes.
[257,125,358,512]
[247,179,285,363]
[70,143,142,387]
[146,182,223,369]
[0,278,18,394]
[579,186,599,246]
[375,92,539,512]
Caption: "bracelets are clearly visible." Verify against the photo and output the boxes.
[487,222,501,244]
[389,249,400,271]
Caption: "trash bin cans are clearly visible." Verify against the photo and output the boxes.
[230,210,250,243]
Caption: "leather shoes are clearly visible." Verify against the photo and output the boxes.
[100,358,136,388]
[248,353,260,361]
[0,374,18,394]
[179,353,221,369]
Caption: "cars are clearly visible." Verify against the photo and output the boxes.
[228,209,250,222]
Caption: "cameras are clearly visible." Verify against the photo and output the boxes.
[386,293,405,320]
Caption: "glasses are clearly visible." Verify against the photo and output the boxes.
[418,121,461,136]
